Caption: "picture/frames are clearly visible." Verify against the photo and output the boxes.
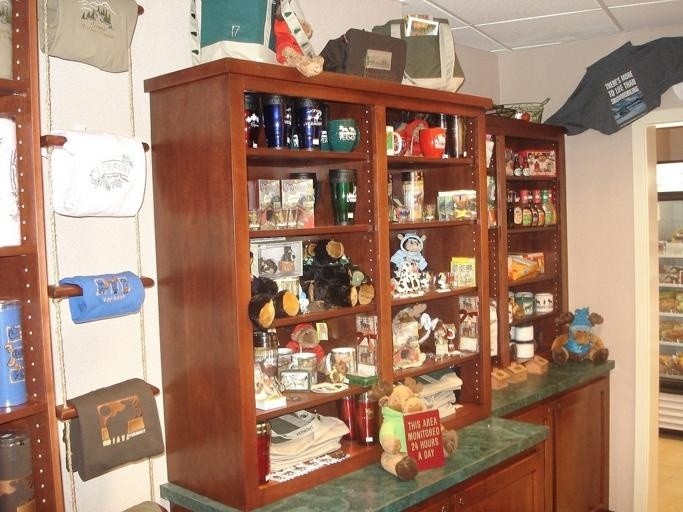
[258,239,303,278]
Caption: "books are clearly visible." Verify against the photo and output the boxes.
[401,409,445,472]
[265,407,350,474]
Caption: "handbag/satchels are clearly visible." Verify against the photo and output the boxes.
[190,0,282,67]
[317,17,463,92]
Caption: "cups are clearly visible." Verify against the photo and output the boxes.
[277,347,292,374]
[330,346,356,373]
[512,322,535,342]
[417,128,446,159]
[533,292,553,314]
[326,119,358,153]
[247,204,301,232]
[385,126,401,156]
[327,168,357,226]
[395,206,410,224]
[289,354,317,386]
[422,200,438,222]
[515,291,533,315]
[514,342,535,361]
[290,171,318,209]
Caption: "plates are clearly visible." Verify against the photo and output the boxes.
[310,381,349,395]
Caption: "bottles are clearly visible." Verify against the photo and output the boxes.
[339,393,378,447]
[387,172,394,222]
[243,91,329,154]
[504,150,531,177]
[252,333,281,388]
[505,188,558,228]
[255,419,271,486]
[444,115,467,159]
[400,169,423,222]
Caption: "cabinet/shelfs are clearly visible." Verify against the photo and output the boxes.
[143,58,492,512]
[477,113,567,372]
[500,375,609,510]
[651,185,683,438]
[402,450,544,512]
